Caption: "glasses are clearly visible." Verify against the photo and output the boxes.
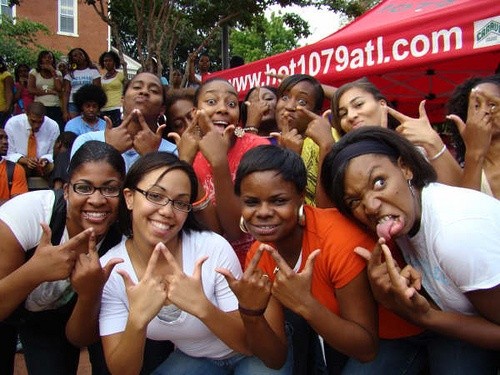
[133,185,194,214]
[69,181,124,198]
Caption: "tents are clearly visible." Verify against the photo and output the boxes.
[202,0,500,126]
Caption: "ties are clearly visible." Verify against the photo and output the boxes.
[28,128,37,158]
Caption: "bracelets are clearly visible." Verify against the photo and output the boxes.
[243,126,259,134]
[41,84,48,94]
[190,190,210,212]
[428,144,446,160]
[237,303,266,317]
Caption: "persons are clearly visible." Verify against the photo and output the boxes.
[320,126,500,351]
[0,48,350,375]
[168,77,273,270]
[98,151,293,375]
[215,144,485,375]
[446,76,500,199]
[331,77,463,187]
[71,72,178,375]
[0,140,126,375]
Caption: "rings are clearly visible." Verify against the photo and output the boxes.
[490,106,495,114]
[262,274,270,278]
[272,266,280,275]
